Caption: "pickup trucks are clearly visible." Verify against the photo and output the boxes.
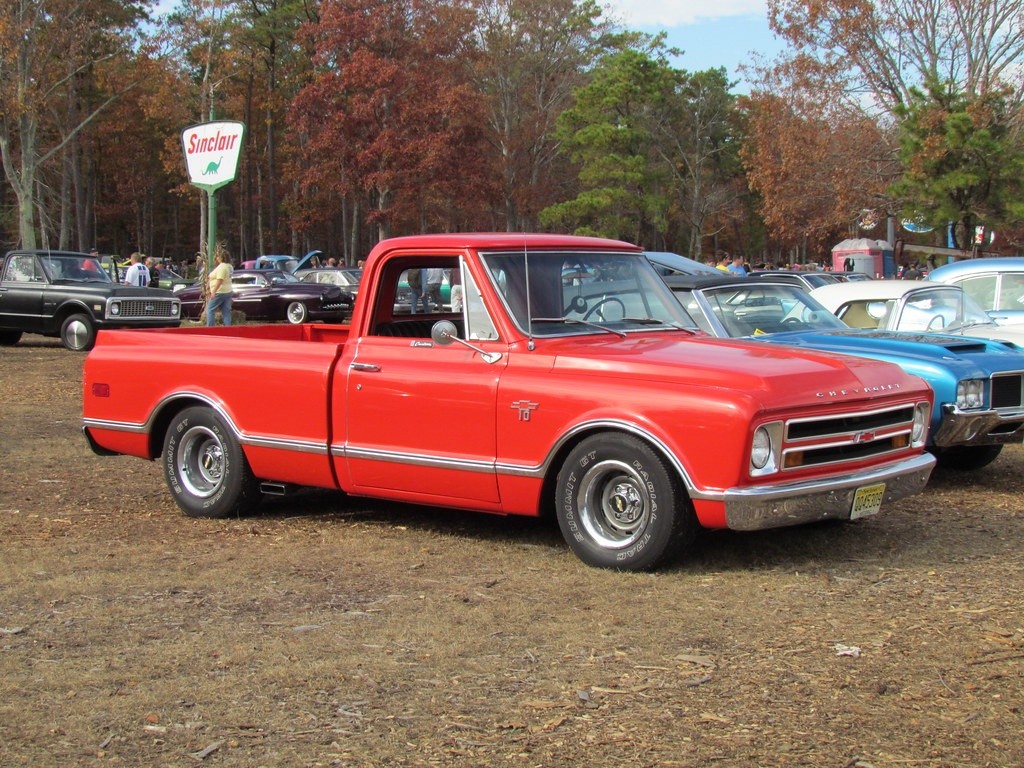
[0,251,182,351]
[82,229,939,569]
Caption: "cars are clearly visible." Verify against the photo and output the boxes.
[644,251,873,328]
[560,266,608,284]
[294,267,436,314]
[779,279,1024,348]
[240,250,324,277]
[100,253,199,292]
[927,256,1024,329]
[398,267,452,303]
[570,280,1024,462]
[173,269,355,324]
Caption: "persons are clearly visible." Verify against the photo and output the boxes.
[260,256,273,269]
[752,258,831,270]
[205,249,234,327]
[901,263,923,280]
[408,268,463,314]
[703,249,747,278]
[83,248,161,289]
[310,252,368,269]
